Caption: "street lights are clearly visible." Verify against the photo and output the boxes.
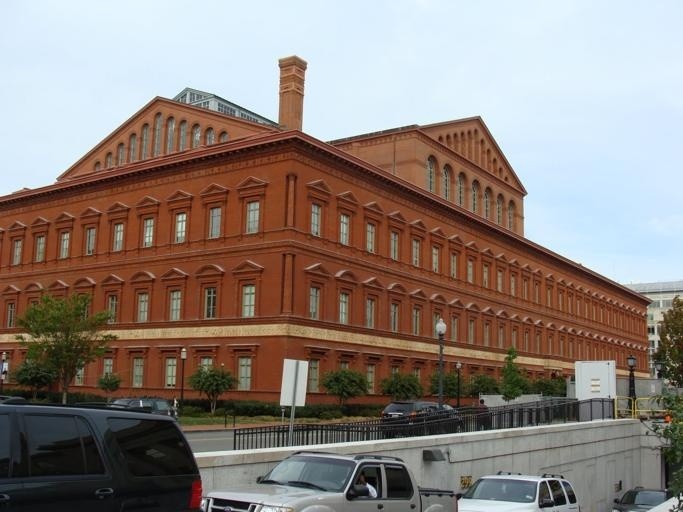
[0,352,6,394]
[455,359,461,409]
[179,346,186,407]
[625,354,637,412]
[435,318,447,411]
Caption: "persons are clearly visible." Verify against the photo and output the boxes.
[341,472,377,500]
[475,399,489,430]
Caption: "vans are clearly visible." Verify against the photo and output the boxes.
[381,400,461,435]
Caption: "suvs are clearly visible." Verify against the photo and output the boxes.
[457,470,580,511]
[0,397,202,512]
[107,398,175,419]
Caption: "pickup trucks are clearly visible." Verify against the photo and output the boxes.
[198,450,457,512]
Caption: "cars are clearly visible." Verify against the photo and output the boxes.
[609,487,672,512]
